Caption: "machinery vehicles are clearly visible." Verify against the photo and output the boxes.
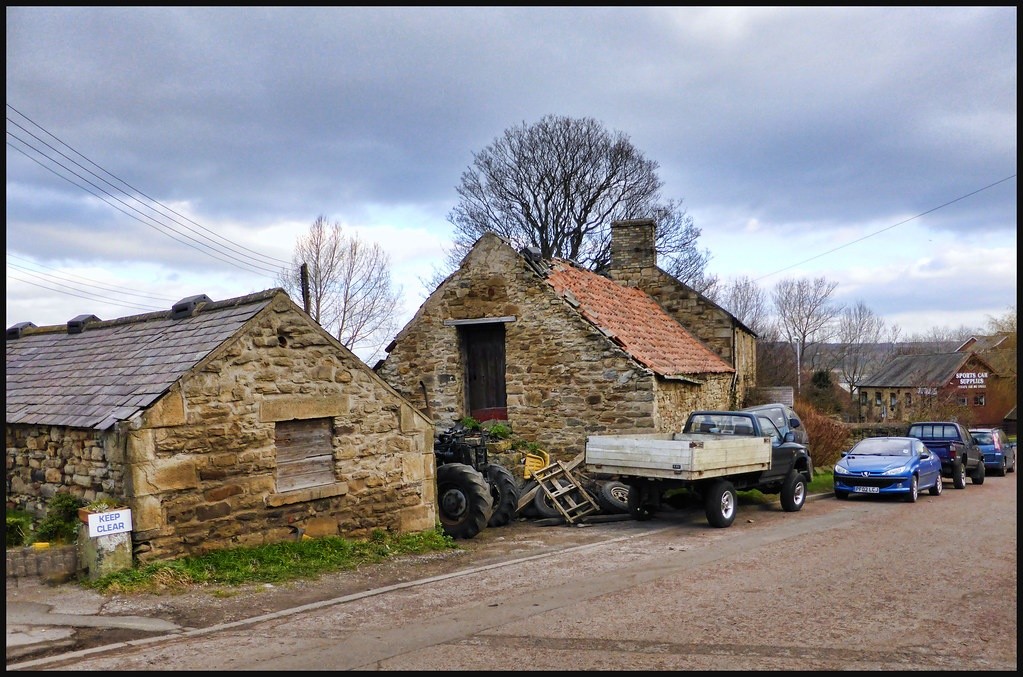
[419,380,518,539]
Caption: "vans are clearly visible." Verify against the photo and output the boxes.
[731,403,814,483]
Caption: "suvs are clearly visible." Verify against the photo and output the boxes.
[968,427,1016,477]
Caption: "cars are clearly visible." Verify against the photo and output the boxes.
[832,436,943,503]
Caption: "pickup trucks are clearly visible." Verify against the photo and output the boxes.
[906,422,986,489]
[584,411,813,529]
